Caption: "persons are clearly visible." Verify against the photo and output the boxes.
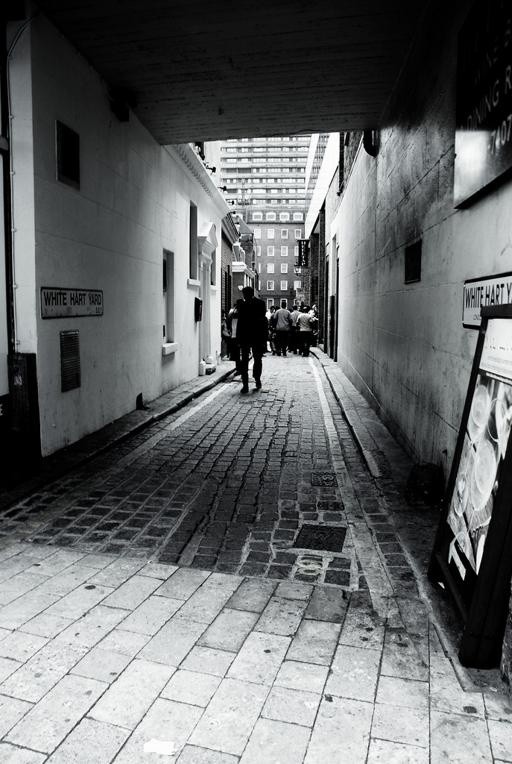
[221,304,237,358]
[228,299,245,376]
[262,301,318,356]
[231,286,268,393]
